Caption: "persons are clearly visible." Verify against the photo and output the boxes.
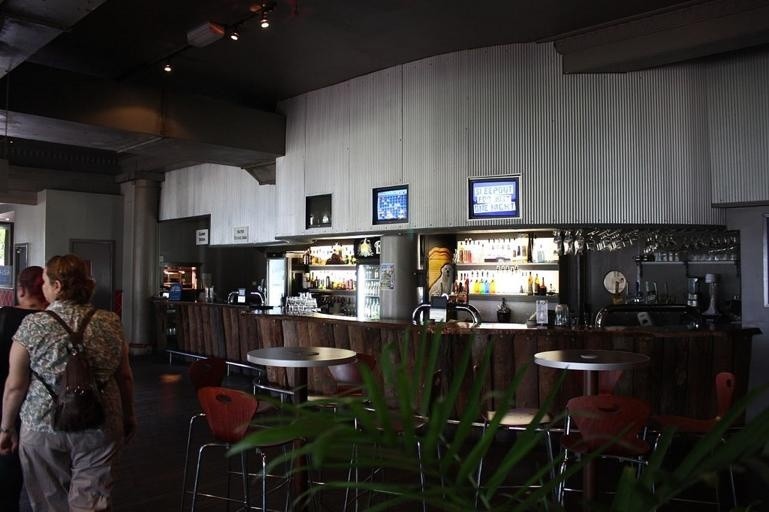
[1,266,50,512]
[0,255,137,512]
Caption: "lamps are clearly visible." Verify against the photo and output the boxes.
[260,12,270,29]
[230,30,240,41]
[163,64,172,72]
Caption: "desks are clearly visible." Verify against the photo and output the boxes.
[534,348,651,502]
[246,345,357,512]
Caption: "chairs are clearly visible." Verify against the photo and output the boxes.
[343,368,443,512]
[651,372,737,510]
[191,386,318,512]
[179,358,289,512]
[557,394,651,508]
[472,364,557,509]
[307,352,377,512]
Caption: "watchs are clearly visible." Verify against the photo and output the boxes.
[0,426,18,433]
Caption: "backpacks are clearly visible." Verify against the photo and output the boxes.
[28,306,119,432]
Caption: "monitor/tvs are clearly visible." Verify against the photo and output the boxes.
[373,185,408,223]
[469,178,518,219]
[305,194,332,229]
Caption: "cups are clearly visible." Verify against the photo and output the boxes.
[583,312,593,330]
[286,292,318,316]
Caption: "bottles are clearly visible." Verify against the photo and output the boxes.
[533,274,540,295]
[467,239,473,264]
[527,272,533,296]
[484,271,490,294]
[540,277,547,296]
[474,272,480,294]
[458,242,463,264]
[490,274,495,293]
[480,272,485,294]
[463,238,468,264]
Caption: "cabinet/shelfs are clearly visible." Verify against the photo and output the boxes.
[154,297,764,432]
[451,231,561,302]
[354,236,381,319]
[300,239,358,318]
